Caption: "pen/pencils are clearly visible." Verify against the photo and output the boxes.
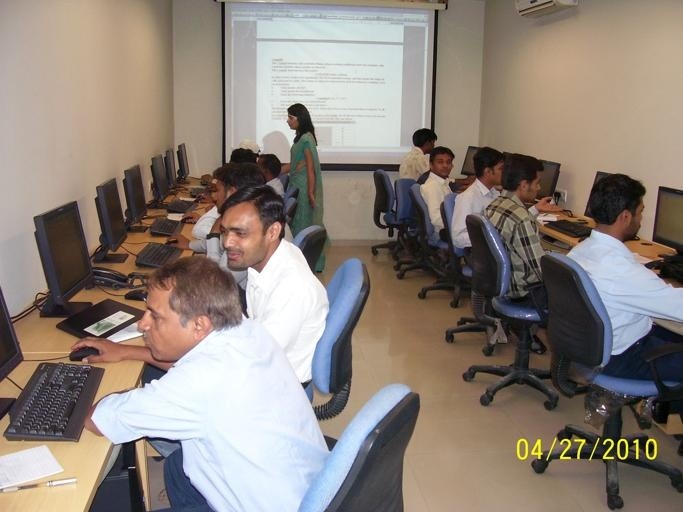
[0,478,77,493]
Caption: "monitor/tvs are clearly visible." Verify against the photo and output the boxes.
[149,142,190,208]
[92,177,129,263]
[123,164,148,232]
[33,200,94,318]
[460,146,683,266]
[0,287,23,420]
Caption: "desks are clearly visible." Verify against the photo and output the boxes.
[3,174,228,354]
[0,354,151,510]
[529,196,682,335]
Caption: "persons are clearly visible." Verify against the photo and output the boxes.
[70,253,331,511]
[478,156,548,309]
[398,127,477,188]
[186,147,284,319]
[450,145,567,264]
[217,184,330,393]
[414,170,476,193]
[280,103,328,274]
[560,172,682,455]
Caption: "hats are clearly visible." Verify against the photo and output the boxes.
[232,140,260,155]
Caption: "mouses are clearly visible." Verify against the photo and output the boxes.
[180,216,196,224]
[165,239,178,245]
[125,290,147,300]
[70,347,99,361]
[194,196,205,202]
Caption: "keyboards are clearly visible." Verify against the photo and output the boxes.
[190,187,211,197]
[136,242,184,268]
[4,363,105,441]
[151,217,184,236]
[643,258,683,286]
[167,199,197,213]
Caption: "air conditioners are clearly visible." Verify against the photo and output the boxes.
[512,0,579,18]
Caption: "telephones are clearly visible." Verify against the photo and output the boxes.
[91,267,130,288]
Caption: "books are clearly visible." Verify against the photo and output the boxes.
[54,297,145,343]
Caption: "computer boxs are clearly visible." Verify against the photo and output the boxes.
[89,441,145,512]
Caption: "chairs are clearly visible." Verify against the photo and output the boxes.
[442,193,546,360]
[278,171,325,273]
[410,184,479,308]
[298,255,368,418]
[369,169,398,260]
[464,211,570,411]
[530,252,678,508]
[392,177,438,279]
[297,382,420,512]
